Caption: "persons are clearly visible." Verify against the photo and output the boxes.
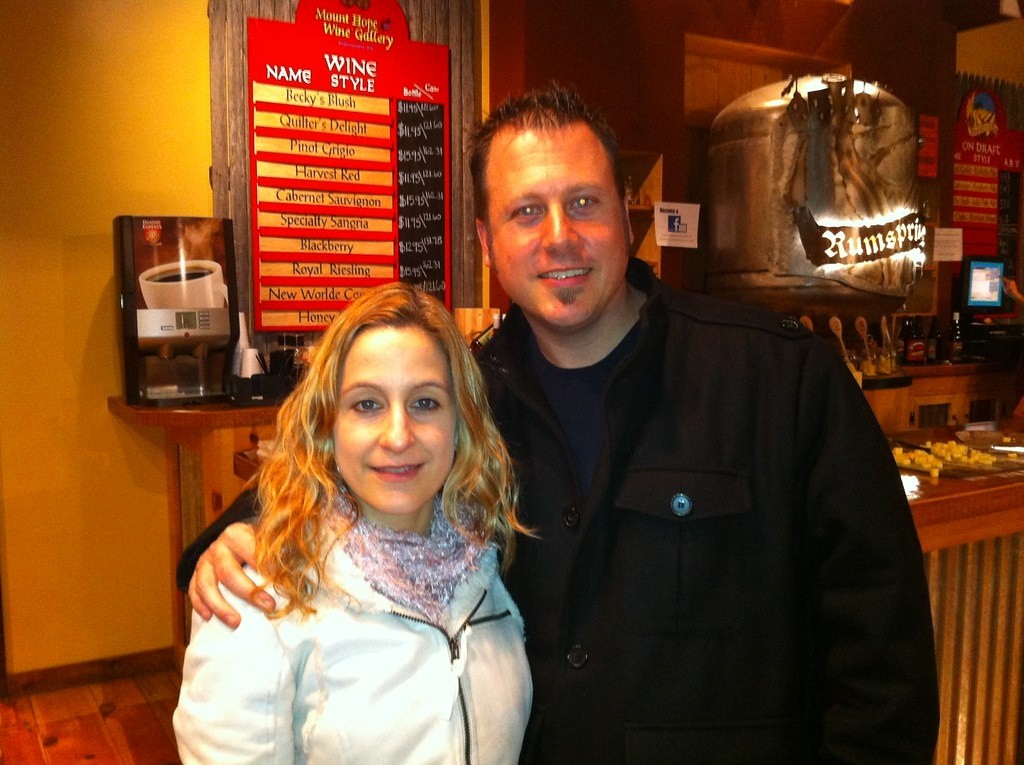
[170,283,537,765]
[997,274,1023,419]
[169,85,940,765]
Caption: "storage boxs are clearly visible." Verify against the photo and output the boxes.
[703,74,928,323]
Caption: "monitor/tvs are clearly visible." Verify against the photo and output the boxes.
[960,255,1011,314]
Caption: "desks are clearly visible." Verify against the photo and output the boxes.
[856,347,1023,385]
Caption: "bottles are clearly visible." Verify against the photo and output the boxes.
[906,315,927,366]
[897,315,910,365]
[269,333,285,401]
[291,334,307,391]
[839,345,898,377]
[946,312,963,363]
[280,332,296,399]
[926,314,943,366]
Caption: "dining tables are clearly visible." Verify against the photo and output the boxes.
[881,418,1024,556]
[107,396,282,679]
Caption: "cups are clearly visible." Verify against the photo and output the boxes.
[138,260,230,313]
[231,311,262,378]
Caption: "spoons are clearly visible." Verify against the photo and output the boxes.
[854,316,871,362]
[828,316,848,355]
[799,315,813,332]
[889,312,897,350]
[880,316,887,354]
